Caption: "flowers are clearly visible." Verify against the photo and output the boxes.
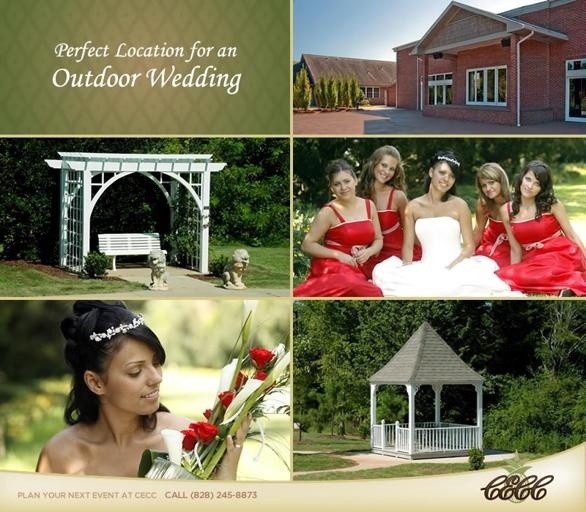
[135,300,291,479]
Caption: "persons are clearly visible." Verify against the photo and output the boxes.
[292,145,586,298]
[35,300,253,479]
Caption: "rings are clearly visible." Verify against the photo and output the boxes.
[234,444,242,448]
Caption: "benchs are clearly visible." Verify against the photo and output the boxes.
[97,232,167,271]
